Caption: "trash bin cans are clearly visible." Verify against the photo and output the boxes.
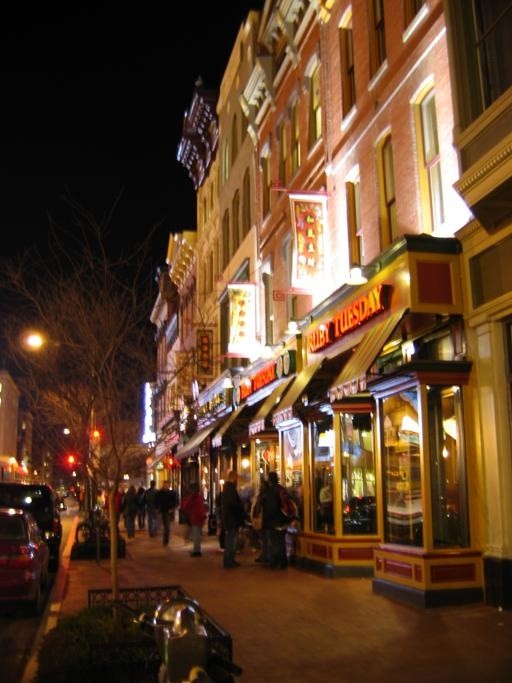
[209,514,217,535]
[285,528,297,563]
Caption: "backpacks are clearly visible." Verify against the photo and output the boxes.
[275,486,298,525]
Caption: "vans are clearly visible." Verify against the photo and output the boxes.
[0,481,67,561]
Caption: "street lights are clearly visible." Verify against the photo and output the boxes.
[28,335,97,449]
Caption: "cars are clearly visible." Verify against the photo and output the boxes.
[0,507,50,620]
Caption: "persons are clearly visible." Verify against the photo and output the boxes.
[70,486,122,533]
[296,479,304,531]
[188,483,207,557]
[215,492,226,549]
[155,480,179,547]
[137,488,147,529]
[221,470,246,567]
[319,478,333,533]
[142,481,159,537]
[254,480,271,563]
[122,486,139,539]
[252,472,292,569]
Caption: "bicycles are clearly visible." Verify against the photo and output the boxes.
[75,515,111,544]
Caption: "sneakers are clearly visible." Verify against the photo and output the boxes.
[190,551,202,557]
[125,523,170,545]
[255,554,289,571]
[222,560,241,569]
